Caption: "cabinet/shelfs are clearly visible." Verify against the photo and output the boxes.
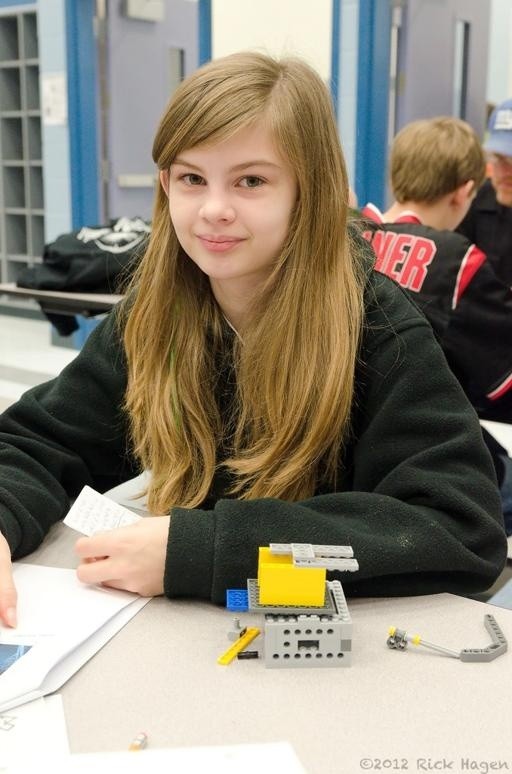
[0,0,44,290]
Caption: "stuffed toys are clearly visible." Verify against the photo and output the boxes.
[218,539,510,669]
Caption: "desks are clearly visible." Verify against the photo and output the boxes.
[0,284,127,317]
[3,521,511,774]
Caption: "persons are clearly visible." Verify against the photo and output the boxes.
[1,46,512,633]
[323,112,511,424]
[454,100,512,281]
